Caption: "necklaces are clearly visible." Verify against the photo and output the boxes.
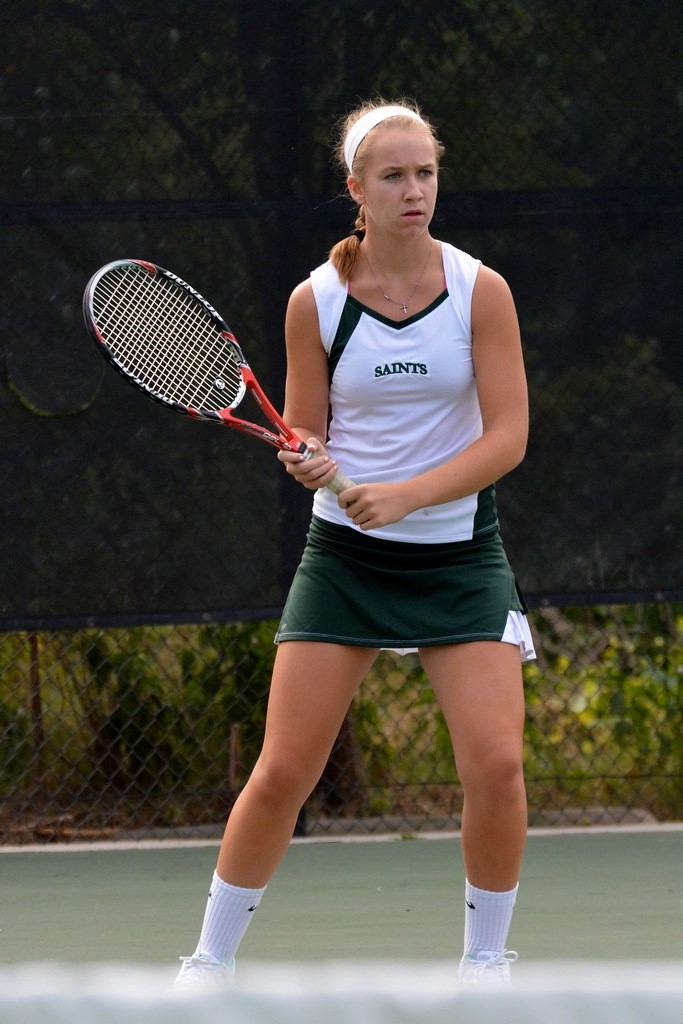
[362,236,433,315]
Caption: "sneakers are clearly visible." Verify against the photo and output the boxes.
[168,951,235,1000]
[458,950,519,1000]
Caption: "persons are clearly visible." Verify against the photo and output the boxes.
[166,97,539,1001]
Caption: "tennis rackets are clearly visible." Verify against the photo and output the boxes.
[84,258,359,502]
